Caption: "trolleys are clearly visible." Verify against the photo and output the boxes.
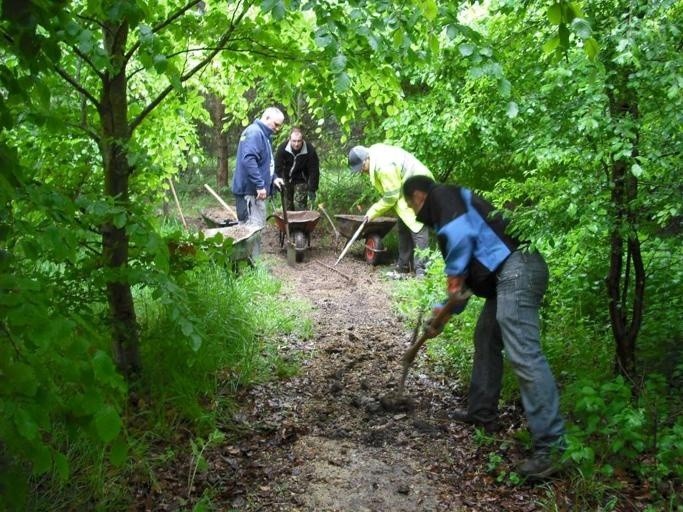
[315,200,398,265]
[196,203,239,230]
[189,219,267,277]
[267,194,323,265]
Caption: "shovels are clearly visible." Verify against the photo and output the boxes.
[278,185,296,268]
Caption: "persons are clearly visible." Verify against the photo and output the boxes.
[400,173,579,482]
[230,106,286,256]
[274,127,319,211]
[346,143,439,282]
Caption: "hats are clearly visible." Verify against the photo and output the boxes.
[349,145,368,169]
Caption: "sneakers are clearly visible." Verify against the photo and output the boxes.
[518,450,573,478]
[386,271,412,280]
[454,408,499,432]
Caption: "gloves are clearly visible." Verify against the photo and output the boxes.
[423,304,452,338]
[308,192,316,200]
[448,276,473,306]
